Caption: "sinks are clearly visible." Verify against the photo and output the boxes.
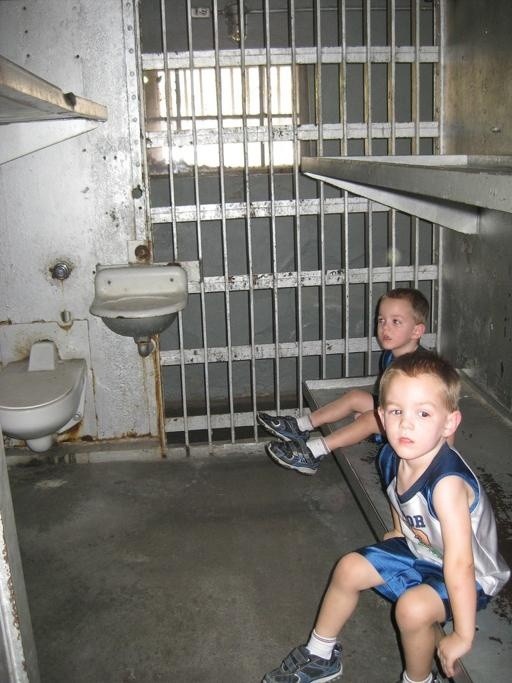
[89,296,189,337]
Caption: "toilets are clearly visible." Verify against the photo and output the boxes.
[0,341,86,452]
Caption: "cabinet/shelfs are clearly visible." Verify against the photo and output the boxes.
[298,153,512,683]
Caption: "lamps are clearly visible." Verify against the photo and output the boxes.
[218,0,249,42]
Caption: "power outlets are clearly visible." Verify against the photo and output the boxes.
[191,8,210,18]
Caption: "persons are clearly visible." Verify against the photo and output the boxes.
[254,287,432,475]
[259,348,511,682]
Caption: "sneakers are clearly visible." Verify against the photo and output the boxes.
[266,438,319,476]
[261,644,343,683]
[257,413,310,444]
